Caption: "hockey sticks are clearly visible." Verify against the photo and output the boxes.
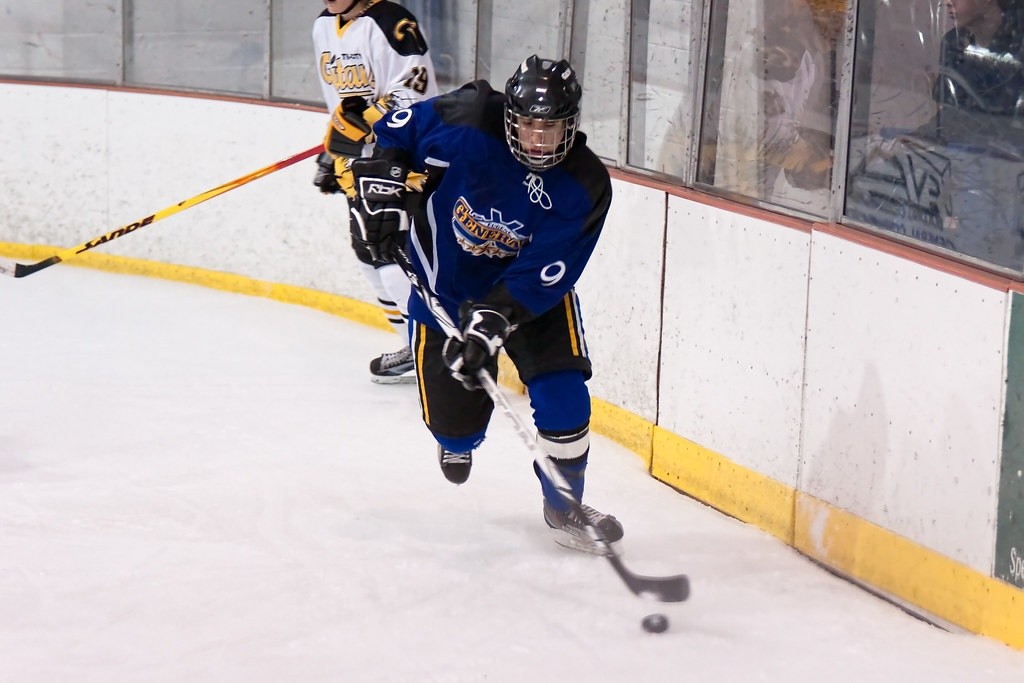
[0,142,325,280]
[388,244,690,604]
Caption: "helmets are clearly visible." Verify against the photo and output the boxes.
[505,54,582,128]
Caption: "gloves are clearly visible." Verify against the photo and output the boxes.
[350,158,410,269]
[784,142,832,190]
[324,95,372,160]
[313,152,342,196]
[441,299,521,391]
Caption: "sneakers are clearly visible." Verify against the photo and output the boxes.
[543,498,624,556]
[370,345,417,385]
[437,443,472,484]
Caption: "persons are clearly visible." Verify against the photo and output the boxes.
[658,0,847,211]
[352,55,628,558]
[312,0,436,384]
[883,0,1024,268]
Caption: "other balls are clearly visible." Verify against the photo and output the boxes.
[643,613,671,634]
